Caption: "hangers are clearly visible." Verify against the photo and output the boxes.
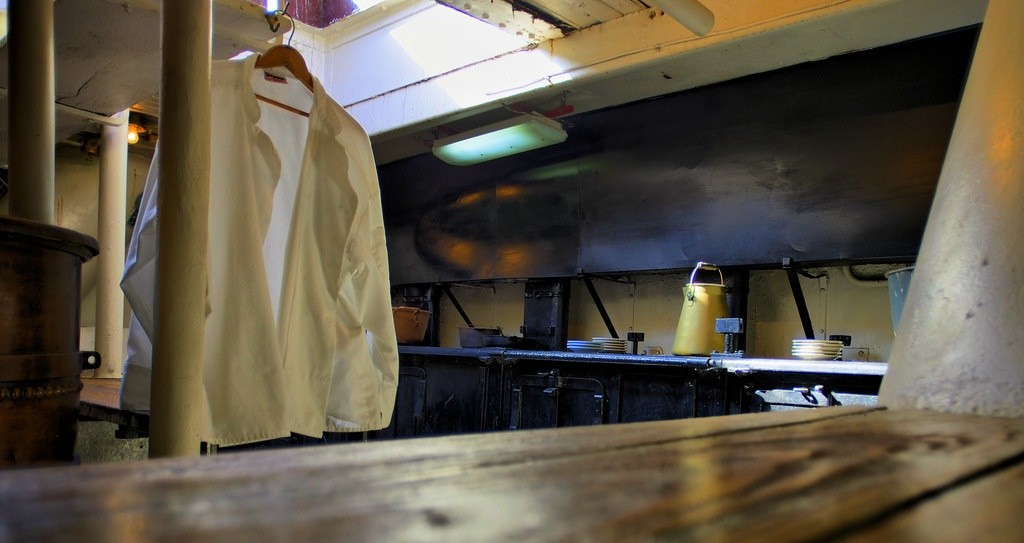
[254,9,313,117]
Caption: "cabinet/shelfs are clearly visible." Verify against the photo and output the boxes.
[299,345,889,447]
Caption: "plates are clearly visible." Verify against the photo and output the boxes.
[566,337,627,353]
[791,339,843,359]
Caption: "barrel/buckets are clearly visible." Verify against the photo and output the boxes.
[885,265,915,334]
[392,306,432,343]
[671,260,729,355]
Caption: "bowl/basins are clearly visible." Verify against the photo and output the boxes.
[459,325,502,348]
[482,334,510,348]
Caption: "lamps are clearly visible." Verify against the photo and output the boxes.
[431,90,571,167]
